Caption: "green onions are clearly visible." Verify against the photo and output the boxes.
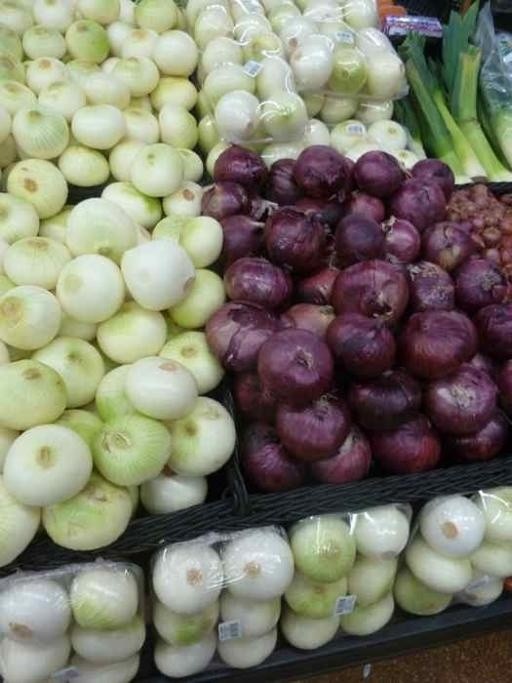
[391,1,512,183]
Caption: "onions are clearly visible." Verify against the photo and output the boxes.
[0,0,512,683]
